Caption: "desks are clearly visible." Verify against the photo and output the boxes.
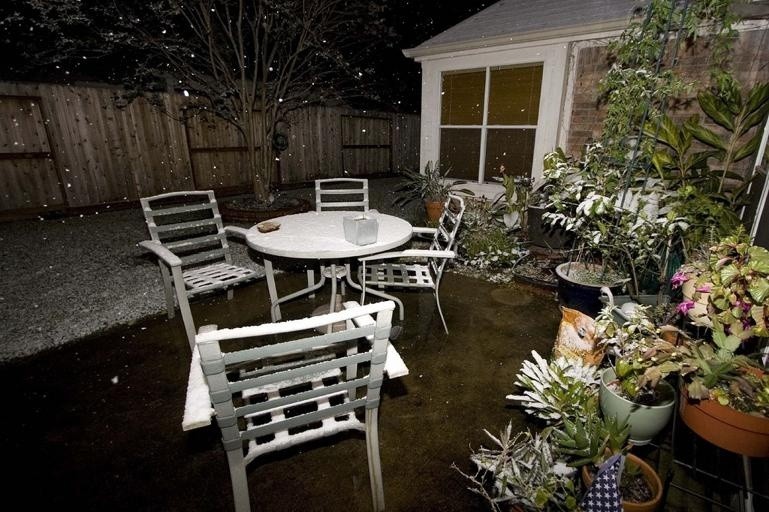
[246,210,404,321]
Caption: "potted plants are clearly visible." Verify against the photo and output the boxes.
[529,154,573,249]
[582,415,663,511]
[557,5,727,315]
[684,333,769,458]
[388,160,475,222]
[599,319,682,447]
[600,158,675,327]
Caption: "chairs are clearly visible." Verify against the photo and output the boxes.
[358,195,464,335]
[140,191,282,353]
[316,179,370,294]
[180,298,410,511]
